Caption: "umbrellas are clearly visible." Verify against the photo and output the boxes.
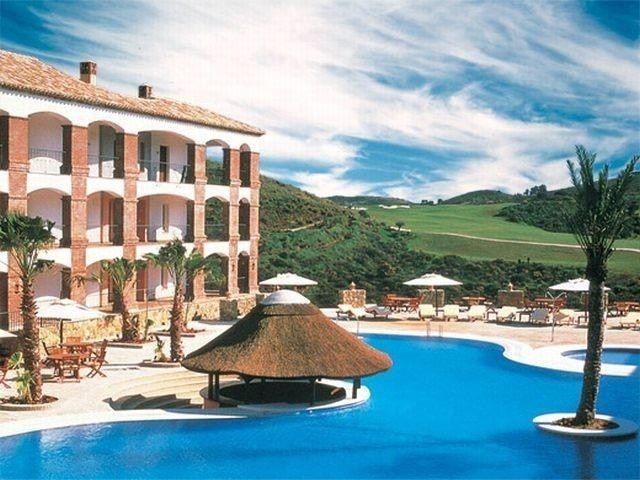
[548,277,611,322]
[258,270,320,289]
[35,294,119,349]
[402,271,463,317]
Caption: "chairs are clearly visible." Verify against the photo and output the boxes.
[335,293,640,331]
[0,337,112,391]
